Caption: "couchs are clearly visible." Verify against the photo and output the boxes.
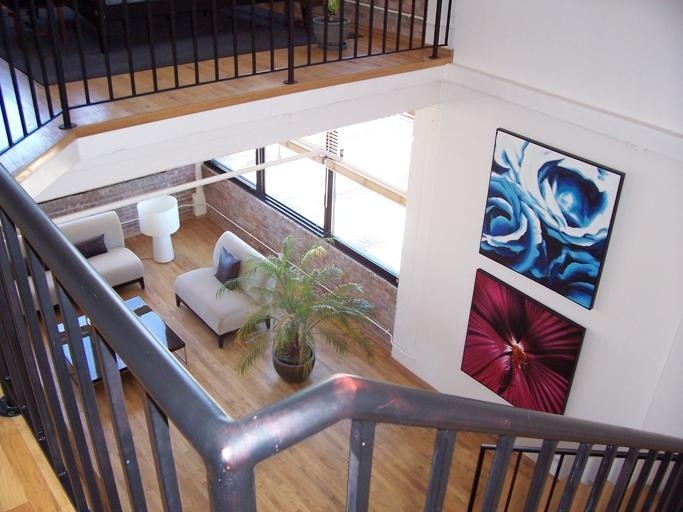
[63,0,313,55]
[174,230,279,348]
[0,210,145,317]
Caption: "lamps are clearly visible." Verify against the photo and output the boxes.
[137,195,181,264]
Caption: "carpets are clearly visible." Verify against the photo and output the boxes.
[0,0,364,87]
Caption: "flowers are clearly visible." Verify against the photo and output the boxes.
[460,269,585,416]
[478,128,626,311]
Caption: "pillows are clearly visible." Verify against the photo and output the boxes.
[214,246,242,291]
[8,254,49,280]
[74,233,109,259]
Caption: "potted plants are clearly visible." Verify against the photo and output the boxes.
[312,0,352,51]
[215,230,378,384]
